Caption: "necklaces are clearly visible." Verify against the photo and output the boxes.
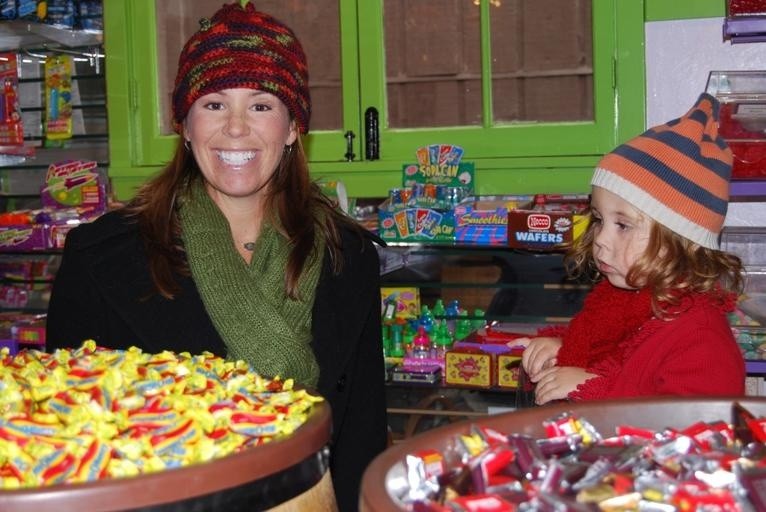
[234,237,258,252]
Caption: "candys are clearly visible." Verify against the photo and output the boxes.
[403,403,765,512]
[1,340,325,488]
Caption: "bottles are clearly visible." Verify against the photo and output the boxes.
[383,299,487,362]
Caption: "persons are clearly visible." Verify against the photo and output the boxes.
[43,1,389,512]
[505,90,749,407]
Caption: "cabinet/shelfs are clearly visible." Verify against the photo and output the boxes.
[3,204,761,398]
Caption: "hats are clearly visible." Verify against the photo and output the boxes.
[589,93,734,250]
[173,2,311,136]
